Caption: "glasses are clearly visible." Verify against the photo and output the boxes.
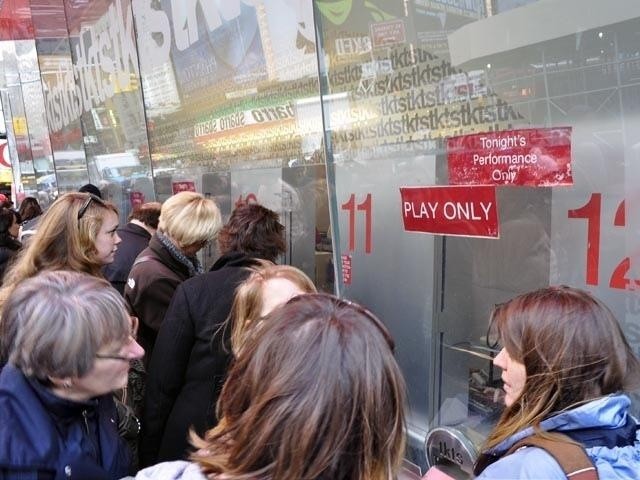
[96,316,140,359]
[78,192,103,218]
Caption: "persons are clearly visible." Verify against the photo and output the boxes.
[470,282,640,478]
[0,184,411,479]
